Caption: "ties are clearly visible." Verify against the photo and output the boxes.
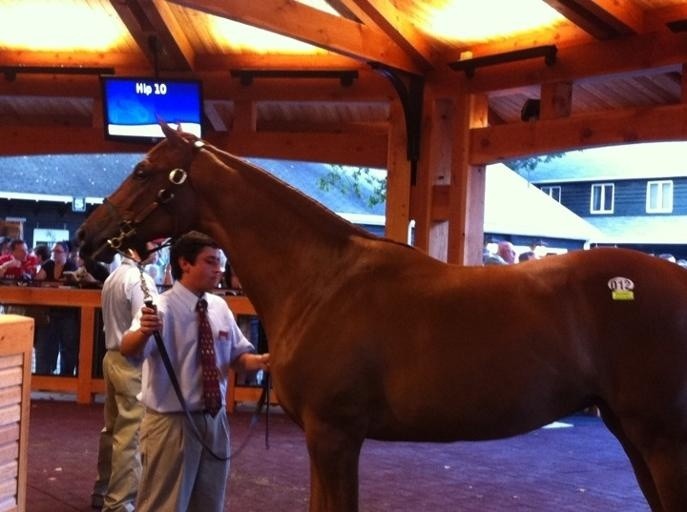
[195,298,223,419]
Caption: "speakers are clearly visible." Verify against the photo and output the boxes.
[521,99,540,121]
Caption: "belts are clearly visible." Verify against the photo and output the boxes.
[146,406,210,416]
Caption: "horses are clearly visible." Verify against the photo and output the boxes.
[74,110,686,512]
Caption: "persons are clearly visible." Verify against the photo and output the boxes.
[484,243,676,280]
[0,236,269,376]
[122,236,270,512]
[89,242,150,512]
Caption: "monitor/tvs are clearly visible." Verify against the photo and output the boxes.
[99,74,206,146]
